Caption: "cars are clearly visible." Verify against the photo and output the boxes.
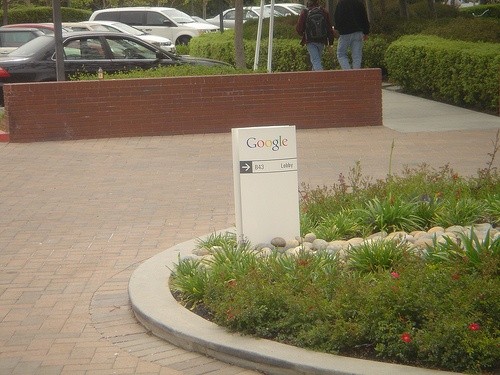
[0,20,176,58]
[0,30,236,107]
[191,2,310,30]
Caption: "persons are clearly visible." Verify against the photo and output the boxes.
[296,0,334,71]
[334,0,369,70]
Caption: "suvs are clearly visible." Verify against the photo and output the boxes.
[89,7,222,54]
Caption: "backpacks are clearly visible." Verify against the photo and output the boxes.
[305,6,327,41]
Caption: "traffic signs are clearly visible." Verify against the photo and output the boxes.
[236,125,298,173]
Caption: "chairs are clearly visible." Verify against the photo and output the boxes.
[82,47,103,59]
